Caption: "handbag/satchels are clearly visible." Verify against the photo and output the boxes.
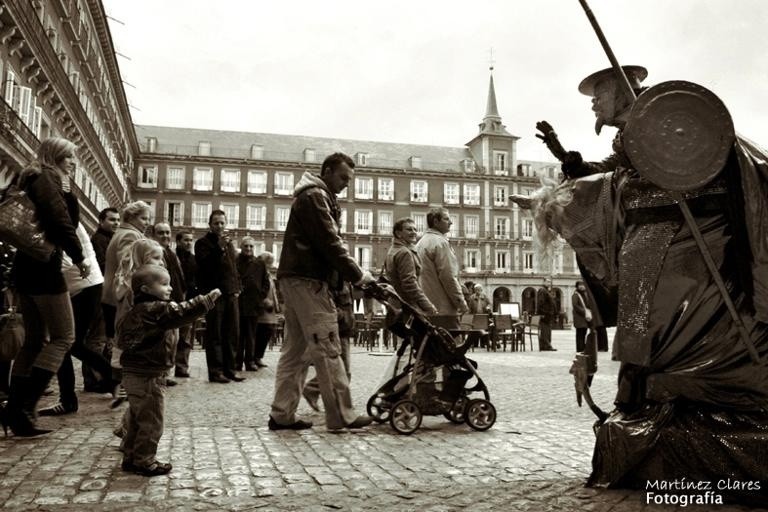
[0,192,55,263]
[0,311,26,361]
[71,326,112,379]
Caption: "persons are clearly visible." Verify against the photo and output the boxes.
[536,278,557,351]
[0,137,494,476]
[535,65,768,412]
[571,280,593,352]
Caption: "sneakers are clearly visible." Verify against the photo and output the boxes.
[267,418,312,430]
[122,460,171,476]
[40,404,70,415]
[332,415,372,430]
[108,381,128,410]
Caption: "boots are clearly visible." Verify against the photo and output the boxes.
[3,367,56,440]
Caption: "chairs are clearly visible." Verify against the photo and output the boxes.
[354,310,543,353]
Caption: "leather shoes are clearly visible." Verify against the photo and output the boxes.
[209,359,267,384]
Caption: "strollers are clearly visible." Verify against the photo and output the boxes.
[361,279,497,435]
